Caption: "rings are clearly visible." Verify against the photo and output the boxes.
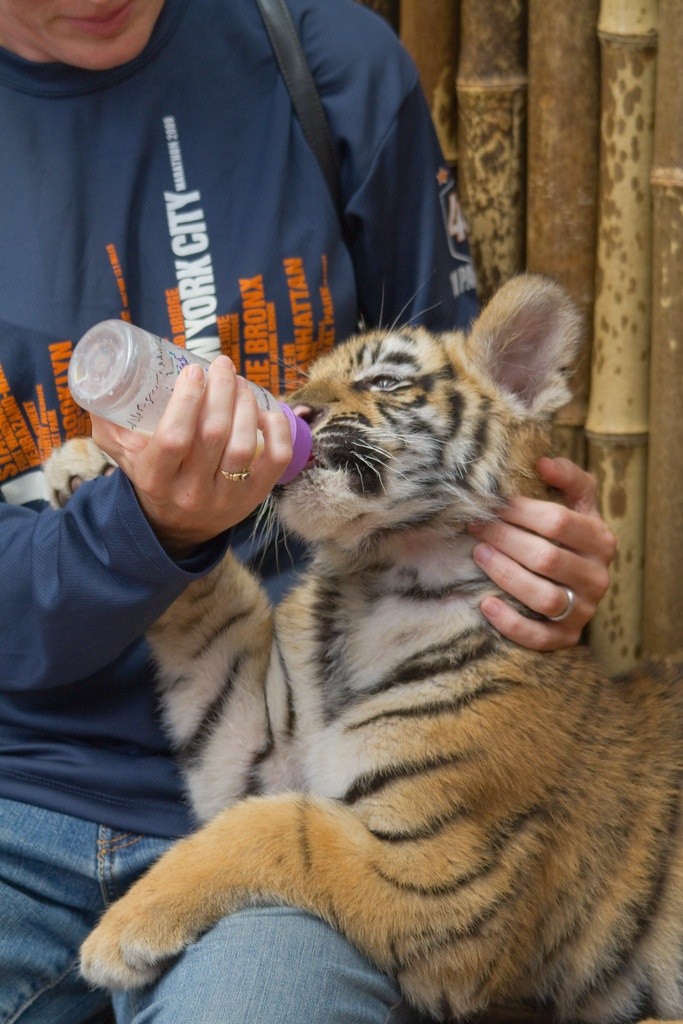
[220,459,256,483]
[545,585,577,624]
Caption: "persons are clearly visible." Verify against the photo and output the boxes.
[0,1,630,1024]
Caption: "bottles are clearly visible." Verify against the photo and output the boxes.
[67,318,314,488]
